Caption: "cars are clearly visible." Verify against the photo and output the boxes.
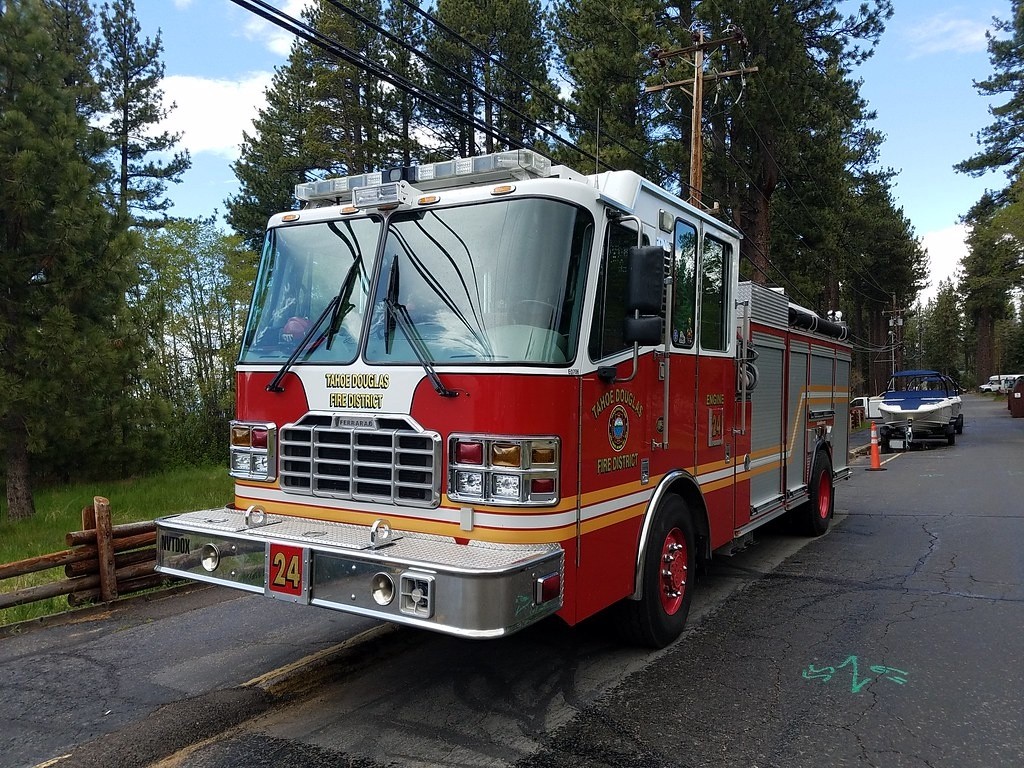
[869,391,893,400]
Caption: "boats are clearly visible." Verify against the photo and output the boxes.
[878,370,953,432]
[907,375,962,418]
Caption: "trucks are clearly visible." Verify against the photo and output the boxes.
[978,375,1024,394]
[849,397,884,418]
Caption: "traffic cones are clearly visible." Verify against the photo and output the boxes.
[865,421,887,472]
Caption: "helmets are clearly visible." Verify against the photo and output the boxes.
[280,317,326,355]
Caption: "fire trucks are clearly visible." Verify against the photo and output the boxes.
[153,146,856,650]
